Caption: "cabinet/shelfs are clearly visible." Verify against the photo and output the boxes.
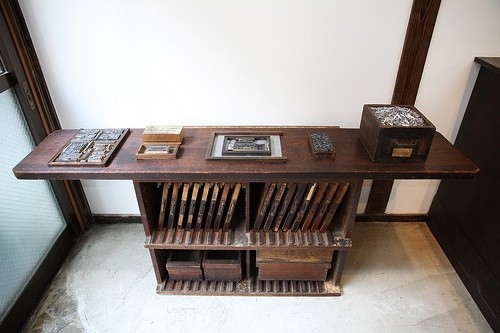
[424,56,500,333]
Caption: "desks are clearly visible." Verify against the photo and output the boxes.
[13,127,478,298]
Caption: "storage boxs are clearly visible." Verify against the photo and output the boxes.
[254,250,332,283]
[202,250,241,282]
[165,251,202,282]
[358,104,437,165]
